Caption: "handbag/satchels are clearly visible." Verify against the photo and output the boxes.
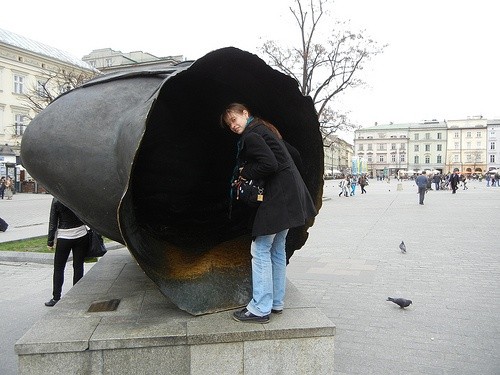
[84,231,106,258]
[232,176,261,209]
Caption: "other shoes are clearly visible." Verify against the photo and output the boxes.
[45,300,55,306]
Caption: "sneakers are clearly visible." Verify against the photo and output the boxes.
[233,307,270,324]
[272,308,281,314]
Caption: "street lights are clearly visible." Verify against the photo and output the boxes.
[331,141,335,179]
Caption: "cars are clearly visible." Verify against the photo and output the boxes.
[323,170,345,180]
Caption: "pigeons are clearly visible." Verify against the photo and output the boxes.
[399,241,406,253]
[386,296,412,310]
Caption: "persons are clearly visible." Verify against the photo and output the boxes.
[221,102,317,325]
[411,168,500,205]
[45,199,107,306]
[0,176,15,200]
[338,172,385,197]
